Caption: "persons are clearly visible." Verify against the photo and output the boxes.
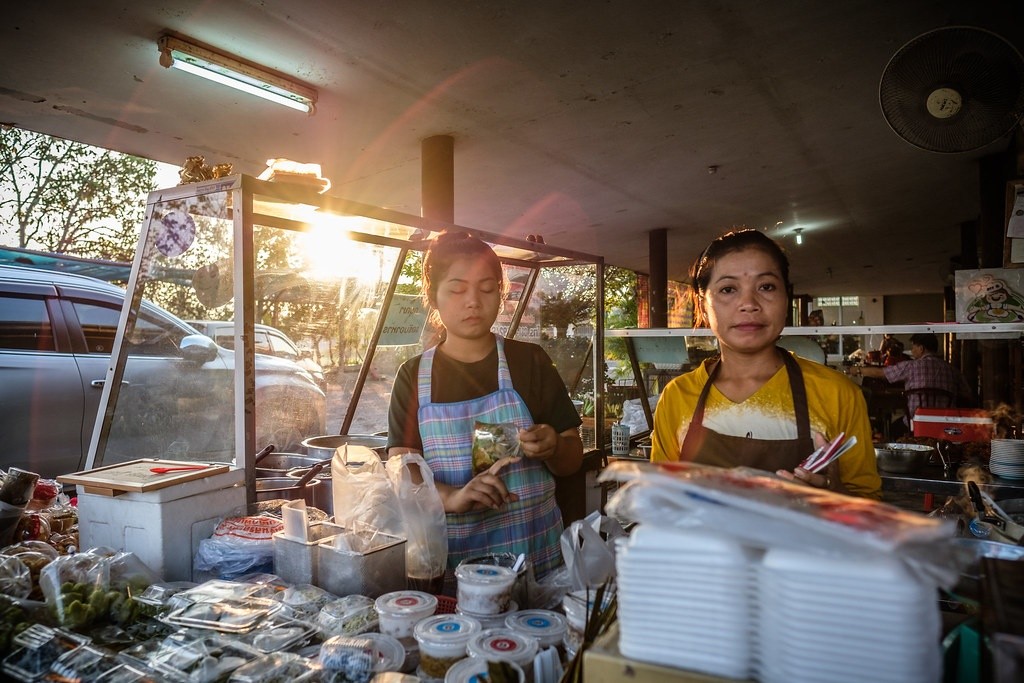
[385,232,584,598]
[840,327,957,443]
[648,227,883,509]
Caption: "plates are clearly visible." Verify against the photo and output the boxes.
[989,438,1024,480]
[614,521,945,683]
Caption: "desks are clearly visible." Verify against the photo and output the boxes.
[597,448,651,543]
[879,462,1024,499]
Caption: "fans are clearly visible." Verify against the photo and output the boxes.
[879,26,1024,154]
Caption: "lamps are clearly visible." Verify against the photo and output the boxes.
[156,35,317,114]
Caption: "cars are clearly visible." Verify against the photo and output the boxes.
[0,263,328,478]
[183,319,325,392]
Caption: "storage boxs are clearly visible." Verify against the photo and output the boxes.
[580,427,615,447]
[911,409,997,443]
[610,385,637,406]
[581,618,741,683]
[0,572,391,683]
[77,462,246,583]
[315,531,406,600]
[271,522,353,586]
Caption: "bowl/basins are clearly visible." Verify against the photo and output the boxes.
[352,565,616,682]
[872,443,935,472]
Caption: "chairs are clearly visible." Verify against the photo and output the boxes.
[902,386,955,436]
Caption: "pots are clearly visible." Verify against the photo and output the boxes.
[232,433,388,512]
